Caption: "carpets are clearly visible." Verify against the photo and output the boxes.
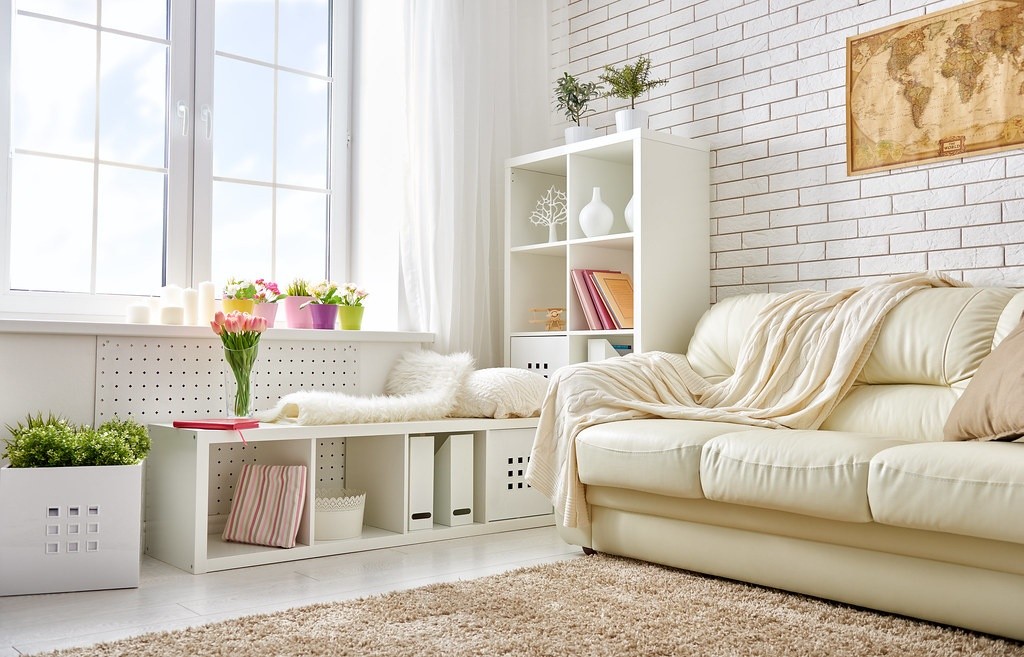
[20,553,1024,657]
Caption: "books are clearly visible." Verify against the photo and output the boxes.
[582,270,621,330]
[571,269,602,331]
[590,271,634,329]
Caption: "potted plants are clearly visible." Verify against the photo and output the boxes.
[0,409,155,597]
[598,57,669,133]
[550,71,605,145]
[298,282,338,329]
[285,278,317,329]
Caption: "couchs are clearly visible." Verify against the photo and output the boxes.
[554,287,1024,641]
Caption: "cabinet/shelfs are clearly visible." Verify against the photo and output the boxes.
[144,417,559,575]
[504,128,710,380]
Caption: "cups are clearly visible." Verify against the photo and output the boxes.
[126,302,149,322]
[198,281,216,326]
[182,288,198,325]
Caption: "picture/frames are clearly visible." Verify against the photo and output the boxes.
[846,0,1024,177]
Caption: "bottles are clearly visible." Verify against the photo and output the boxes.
[579,186,614,238]
[624,194,633,231]
[160,281,184,324]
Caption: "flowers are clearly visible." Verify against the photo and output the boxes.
[222,276,257,300]
[253,278,288,304]
[210,310,268,417]
[337,283,369,306]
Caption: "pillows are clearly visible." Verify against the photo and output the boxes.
[943,312,1024,442]
[446,367,550,419]
[222,463,307,549]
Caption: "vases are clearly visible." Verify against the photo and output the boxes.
[223,341,259,417]
[222,299,255,316]
[624,195,633,232]
[253,303,278,328]
[579,187,614,238]
[338,304,365,330]
